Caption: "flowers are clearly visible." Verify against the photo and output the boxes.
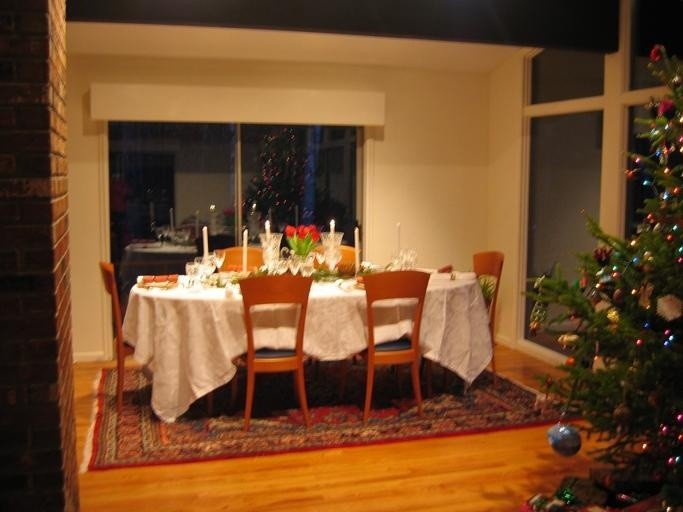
[285,224,321,260]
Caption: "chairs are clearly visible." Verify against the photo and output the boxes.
[217,246,265,272]
[314,244,356,274]
[339,271,431,428]
[472,251,504,388]
[99,261,135,413]
[232,276,311,432]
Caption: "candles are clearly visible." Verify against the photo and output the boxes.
[210,204,216,236]
[243,229,248,272]
[264,220,270,235]
[354,227,360,274]
[330,218,335,234]
[202,226,209,259]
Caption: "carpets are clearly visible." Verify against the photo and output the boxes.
[78,367,583,473]
